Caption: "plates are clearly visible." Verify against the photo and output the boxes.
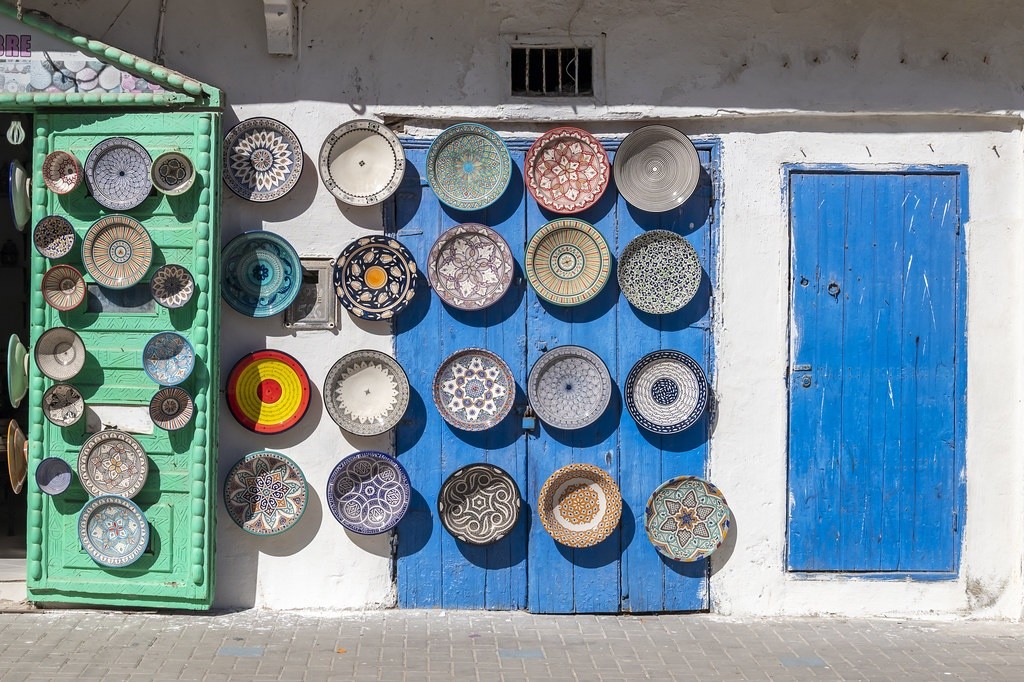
[436,462,522,546]
[617,229,703,315]
[223,450,310,537]
[332,235,420,321]
[426,222,515,311]
[538,463,623,549]
[644,474,731,563]
[224,349,312,435]
[432,347,516,432]
[425,122,512,211]
[318,119,407,206]
[624,349,708,435]
[523,217,612,308]
[220,230,304,318]
[322,349,411,437]
[222,117,304,203]
[527,345,613,430]
[523,126,611,214]
[326,450,412,535]
[612,124,701,212]
[4,137,195,568]
